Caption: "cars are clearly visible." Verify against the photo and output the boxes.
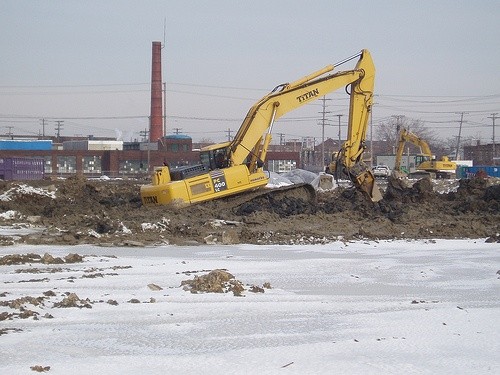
[373,166,392,178]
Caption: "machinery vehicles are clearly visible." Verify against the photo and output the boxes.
[390,129,458,179]
[324,151,343,179]
[139,49,384,217]
